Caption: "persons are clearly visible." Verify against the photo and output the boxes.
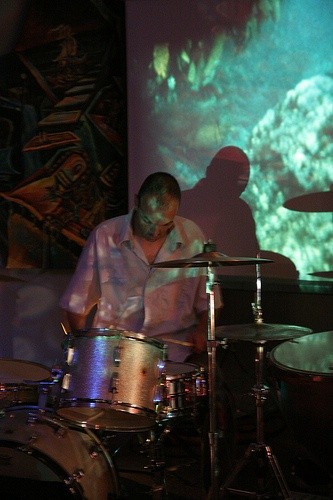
[55,172,224,362]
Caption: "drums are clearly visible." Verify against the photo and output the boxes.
[159,360,208,419]
[0,357,61,414]
[107,408,190,488]
[52,326,169,433]
[0,405,119,500]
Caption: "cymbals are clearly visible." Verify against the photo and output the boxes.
[147,251,276,269]
[213,322,313,341]
[268,330,333,377]
[306,271,333,279]
[281,191,333,213]
